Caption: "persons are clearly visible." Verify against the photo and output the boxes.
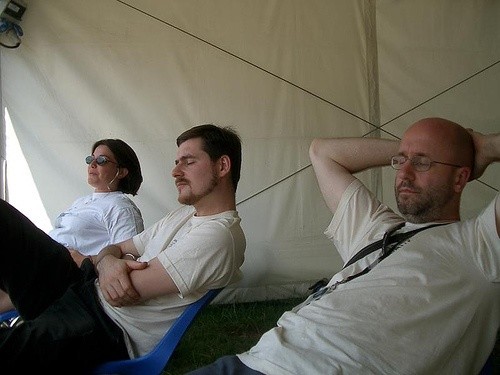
[0,124,246,375]
[0,139,144,313]
[188,118,500,374]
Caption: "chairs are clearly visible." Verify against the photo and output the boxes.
[0,287,226,375]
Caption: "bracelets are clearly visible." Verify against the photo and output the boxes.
[126,252,137,260]
[88,254,94,263]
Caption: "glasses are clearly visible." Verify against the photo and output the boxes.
[85,156,121,168]
[391,154,463,173]
